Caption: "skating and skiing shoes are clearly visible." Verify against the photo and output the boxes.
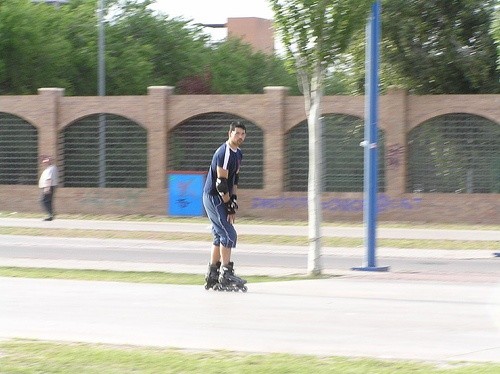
[205,261,222,290]
[213,262,248,292]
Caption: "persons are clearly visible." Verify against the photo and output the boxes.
[38,160,58,221]
[202,121,248,293]
